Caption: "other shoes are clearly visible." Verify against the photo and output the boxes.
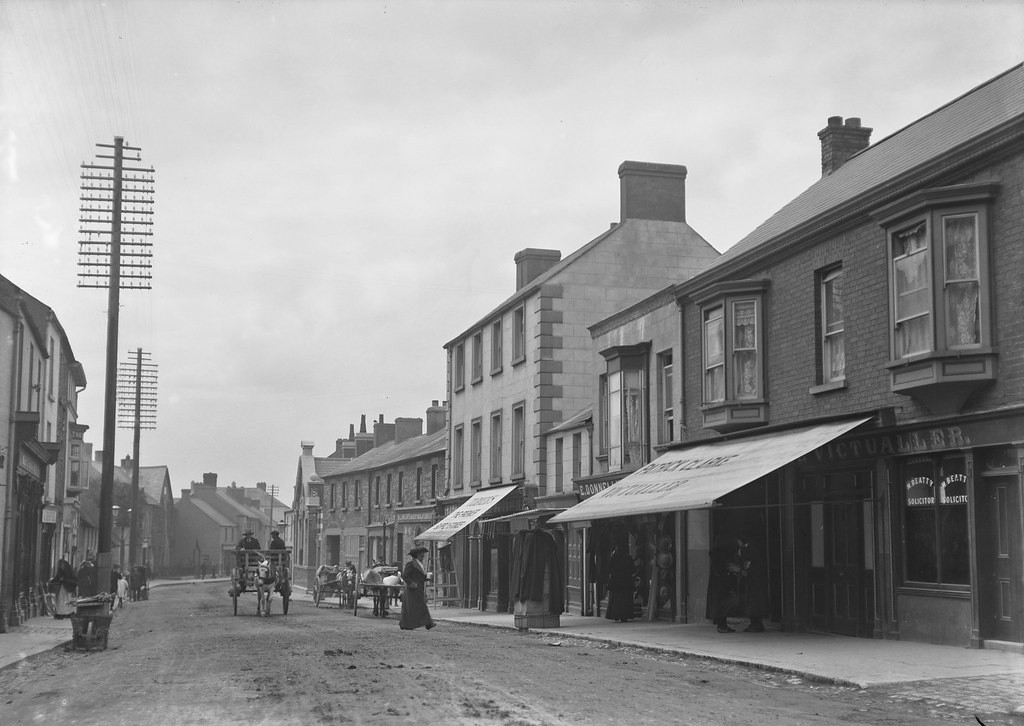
[744,626,764,632]
[718,627,735,633]
[426,623,436,630]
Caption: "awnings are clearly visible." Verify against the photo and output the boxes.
[413,483,519,541]
[479,508,569,522]
[545,410,874,523]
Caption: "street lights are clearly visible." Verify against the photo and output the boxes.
[112,504,132,576]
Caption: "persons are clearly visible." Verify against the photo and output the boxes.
[74,558,96,599]
[200,561,206,580]
[130,568,142,602]
[606,540,633,623]
[709,535,765,633]
[54,558,78,619]
[236,528,261,572]
[399,546,437,630]
[210,561,216,579]
[110,564,130,611]
[332,561,356,583]
[269,530,286,567]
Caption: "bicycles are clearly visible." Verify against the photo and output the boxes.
[40,578,56,615]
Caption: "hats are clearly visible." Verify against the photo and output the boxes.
[656,551,673,570]
[407,547,428,555]
[659,570,674,585]
[657,586,672,601]
[656,535,672,552]
[242,528,254,535]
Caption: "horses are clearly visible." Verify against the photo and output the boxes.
[254,560,276,617]
[337,568,354,609]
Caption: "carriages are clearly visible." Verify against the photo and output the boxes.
[227,547,294,618]
[352,557,406,617]
[306,559,357,608]
